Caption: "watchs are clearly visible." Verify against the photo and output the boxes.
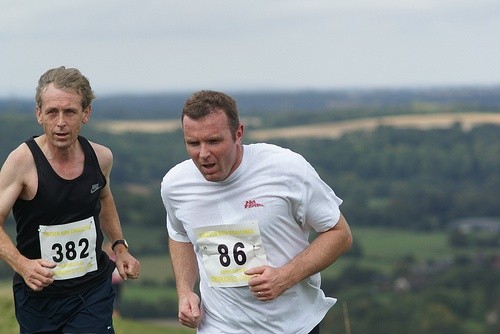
[111,239,129,251]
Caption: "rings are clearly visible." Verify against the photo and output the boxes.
[258,292,262,297]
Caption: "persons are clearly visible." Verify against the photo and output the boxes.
[1,65,141,334]
[161,92,353,334]
[100,243,123,316]
